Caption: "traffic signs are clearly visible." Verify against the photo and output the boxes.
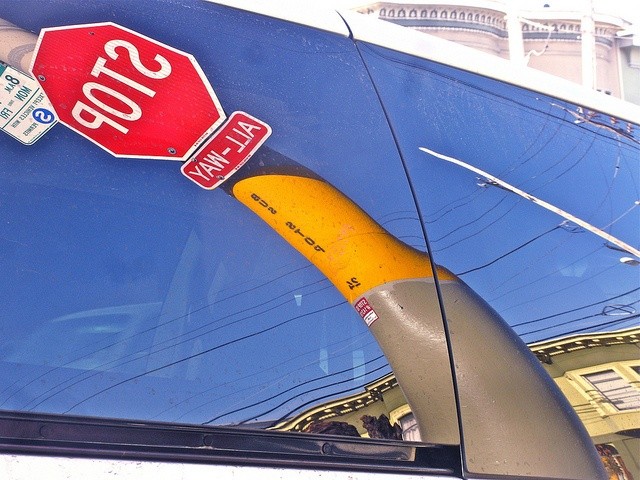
[1,16,599,450]
